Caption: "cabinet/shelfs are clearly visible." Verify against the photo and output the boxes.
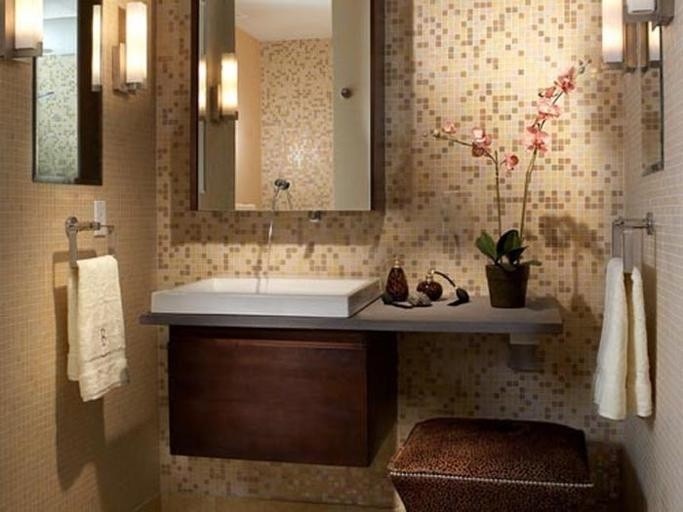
[167,324,399,467]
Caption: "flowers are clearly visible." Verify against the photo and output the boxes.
[422,57,592,273]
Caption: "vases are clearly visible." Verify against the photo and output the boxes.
[485,265,530,309]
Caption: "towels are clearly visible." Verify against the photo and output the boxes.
[591,257,654,420]
[66,255,130,402]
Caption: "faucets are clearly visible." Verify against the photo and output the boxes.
[264,178,289,272]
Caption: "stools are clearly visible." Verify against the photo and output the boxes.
[389,419,592,512]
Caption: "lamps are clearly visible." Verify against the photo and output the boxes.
[113,2,148,96]
[211,51,238,124]
[0,0,44,65]
[601,0,674,75]
[91,4,102,93]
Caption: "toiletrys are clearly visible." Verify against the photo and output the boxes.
[386,254,409,299]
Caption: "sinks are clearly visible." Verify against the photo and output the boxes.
[151,276,381,317]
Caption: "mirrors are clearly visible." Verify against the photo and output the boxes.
[187,0,386,212]
[638,19,664,178]
[32,0,105,186]
